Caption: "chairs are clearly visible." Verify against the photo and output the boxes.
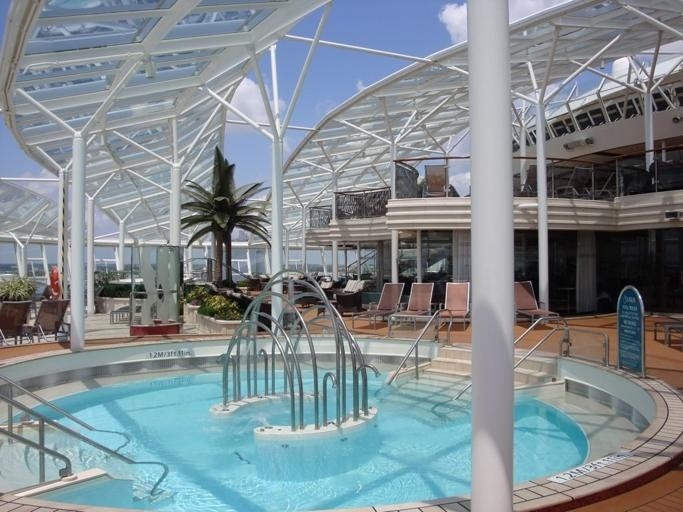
[654,321,683,347]
[514,280,559,329]
[320,280,470,331]
[0,300,32,345]
[20,300,70,343]
[421,164,450,197]
[517,164,615,198]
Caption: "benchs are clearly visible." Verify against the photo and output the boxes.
[110,306,141,325]
